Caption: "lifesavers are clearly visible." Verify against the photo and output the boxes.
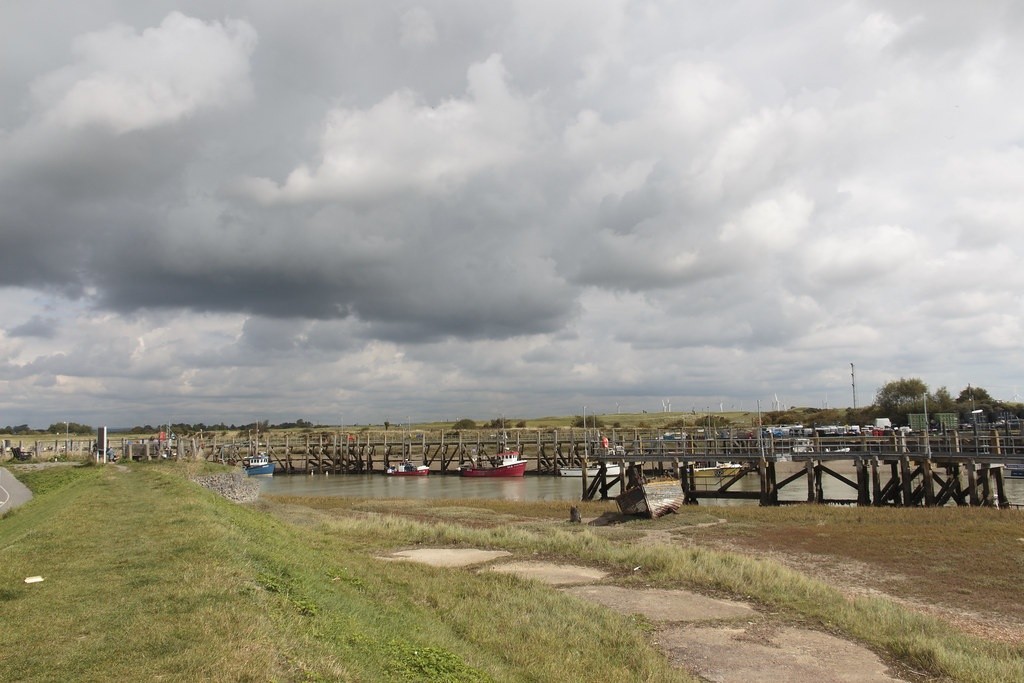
[602,437,609,448]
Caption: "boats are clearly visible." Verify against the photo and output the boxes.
[686,460,743,477]
[384,457,429,477]
[557,461,646,476]
[458,450,528,477]
[241,450,275,478]
